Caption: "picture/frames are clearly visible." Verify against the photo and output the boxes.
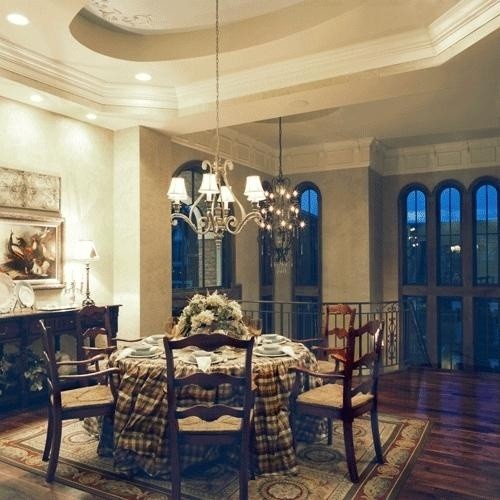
[0,210,67,291]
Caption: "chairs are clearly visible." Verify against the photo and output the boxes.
[159,332,257,500]
[39,319,123,485]
[74,304,144,423]
[285,318,386,485]
[310,304,358,385]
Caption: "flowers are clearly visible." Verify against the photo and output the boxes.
[0,346,47,404]
[169,287,256,340]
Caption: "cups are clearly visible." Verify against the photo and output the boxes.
[193,350,213,362]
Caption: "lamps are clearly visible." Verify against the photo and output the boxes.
[164,0,308,267]
[72,239,101,307]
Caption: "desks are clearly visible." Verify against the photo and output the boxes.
[1,304,123,352]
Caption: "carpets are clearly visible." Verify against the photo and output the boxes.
[1,409,432,500]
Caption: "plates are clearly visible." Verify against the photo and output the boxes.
[125,334,166,357]
[0,271,35,313]
[251,344,291,357]
[179,353,224,364]
